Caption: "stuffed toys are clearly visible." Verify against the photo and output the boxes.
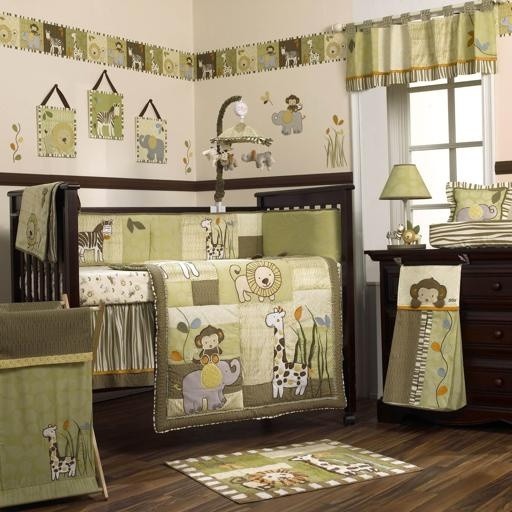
[241,150,275,171]
[203,147,239,172]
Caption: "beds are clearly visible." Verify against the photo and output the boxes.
[5,184,356,430]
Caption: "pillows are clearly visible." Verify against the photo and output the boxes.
[445,181,512,228]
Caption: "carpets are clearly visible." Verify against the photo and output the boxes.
[162,436,422,507]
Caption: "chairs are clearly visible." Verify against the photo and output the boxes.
[0,299,112,508]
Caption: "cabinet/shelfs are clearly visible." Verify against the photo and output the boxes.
[365,243,511,435]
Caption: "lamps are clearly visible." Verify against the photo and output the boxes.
[377,163,433,249]
